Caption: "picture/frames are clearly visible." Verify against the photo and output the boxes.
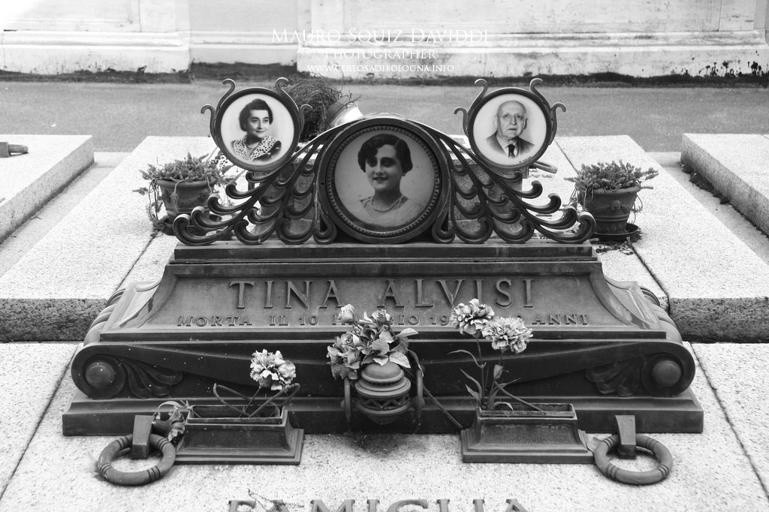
[468,87,552,170]
[215,87,299,173]
[318,118,450,243]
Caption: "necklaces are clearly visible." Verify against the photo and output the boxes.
[368,195,406,212]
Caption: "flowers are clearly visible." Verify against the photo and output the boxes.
[442,297,535,408]
[238,347,301,418]
[325,303,420,382]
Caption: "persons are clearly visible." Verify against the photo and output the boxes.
[349,133,423,225]
[228,99,282,163]
[488,100,534,157]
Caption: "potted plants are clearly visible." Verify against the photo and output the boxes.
[272,70,363,142]
[131,151,221,235]
[572,160,658,240]
